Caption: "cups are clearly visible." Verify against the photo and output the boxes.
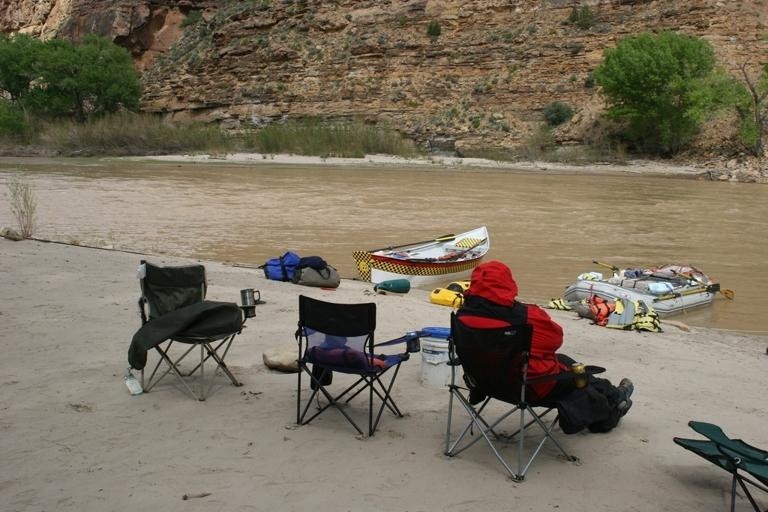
[239,288,261,306]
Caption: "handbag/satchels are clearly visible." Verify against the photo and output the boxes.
[256,250,341,290]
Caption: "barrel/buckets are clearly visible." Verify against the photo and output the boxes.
[420,328,464,387]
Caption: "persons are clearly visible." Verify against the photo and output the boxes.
[457,260,634,434]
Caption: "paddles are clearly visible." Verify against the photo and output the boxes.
[670,268,733,299]
[455,237,487,256]
[367,234,456,254]
[652,283,721,301]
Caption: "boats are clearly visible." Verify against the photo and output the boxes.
[564,263,714,316]
[353,225,490,287]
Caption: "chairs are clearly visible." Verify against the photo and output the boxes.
[137,259,256,401]
[294,293,412,439]
[443,311,605,481]
